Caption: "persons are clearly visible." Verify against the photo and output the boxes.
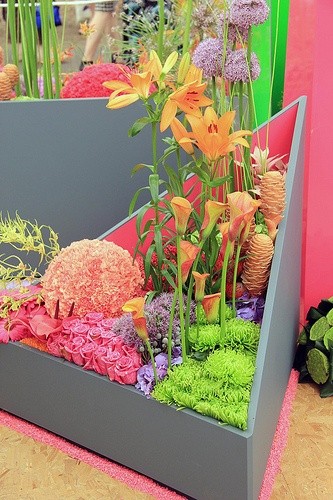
[77,0,125,71]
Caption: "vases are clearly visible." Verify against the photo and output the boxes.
[0,95,307,500]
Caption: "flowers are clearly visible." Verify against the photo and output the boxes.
[0,0,292,430]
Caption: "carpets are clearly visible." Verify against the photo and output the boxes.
[1,300,306,500]
